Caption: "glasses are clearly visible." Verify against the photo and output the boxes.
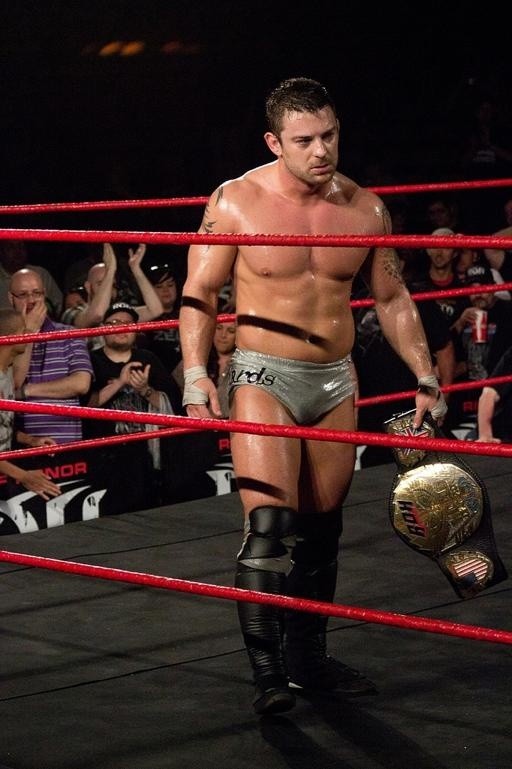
[10,291,41,299]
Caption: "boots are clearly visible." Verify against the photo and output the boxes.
[233,572,378,714]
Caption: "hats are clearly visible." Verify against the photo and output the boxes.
[104,301,138,324]
[465,266,497,285]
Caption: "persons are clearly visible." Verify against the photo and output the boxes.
[178,78,447,713]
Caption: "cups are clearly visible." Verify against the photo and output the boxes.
[469,309,488,345]
